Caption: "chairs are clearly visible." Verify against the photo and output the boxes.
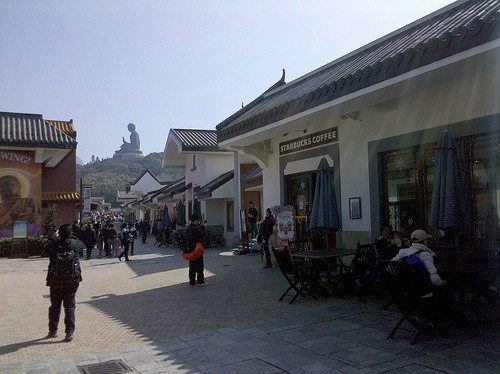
[271,238,468,345]
[153,230,168,247]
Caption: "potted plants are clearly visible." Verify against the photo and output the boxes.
[37,208,60,257]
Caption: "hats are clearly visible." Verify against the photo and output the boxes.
[411,230,432,242]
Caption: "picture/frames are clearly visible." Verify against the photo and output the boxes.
[349,197,362,219]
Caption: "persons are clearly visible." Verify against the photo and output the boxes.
[135,218,164,245]
[373,224,412,260]
[185,214,207,286]
[258,208,274,269]
[42,224,86,340]
[71,212,138,262]
[386,229,457,335]
[44,224,60,243]
[156,218,187,248]
[247,201,259,239]
[120,123,140,151]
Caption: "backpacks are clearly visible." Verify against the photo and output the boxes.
[399,249,433,289]
[52,246,77,283]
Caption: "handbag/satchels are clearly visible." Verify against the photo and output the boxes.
[183,243,204,261]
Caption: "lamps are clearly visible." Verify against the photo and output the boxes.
[284,129,307,137]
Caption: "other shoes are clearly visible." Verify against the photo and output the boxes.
[65,334,73,340]
[48,332,56,338]
[198,282,208,286]
[84,257,88,260]
[125,260,131,261]
[263,265,272,269]
[118,256,121,261]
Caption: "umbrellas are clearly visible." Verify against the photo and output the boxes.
[177,201,186,226]
[192,197,202,219]
[308,157,342,252]
[163,204,170,225]
[427,134,472,246]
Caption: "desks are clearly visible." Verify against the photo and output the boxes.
[290,248,365,296]
